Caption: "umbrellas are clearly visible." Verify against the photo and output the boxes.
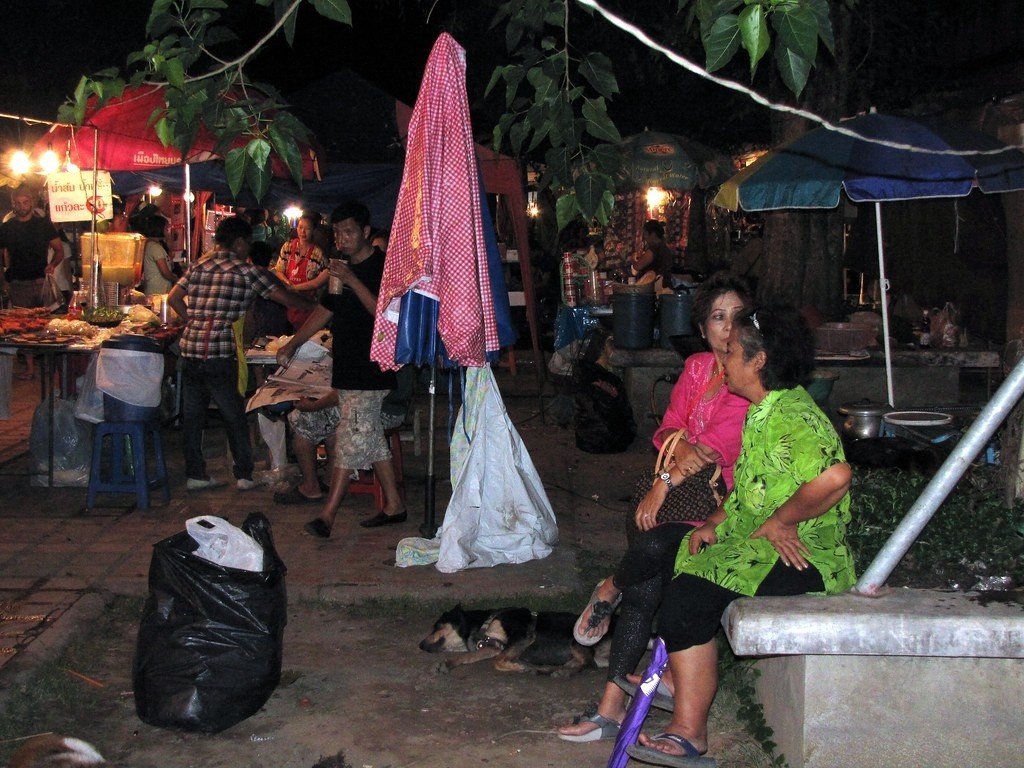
[370,32,519,529]
[711,113,1024,408]
[614,126,738,193]
[41,82,329,264]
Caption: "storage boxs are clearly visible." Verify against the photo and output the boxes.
[80,231,148,287]
[815,321,872,353]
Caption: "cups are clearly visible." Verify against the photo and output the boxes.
[160,301,171,329]
[628,277,636,285]
[327,258,348,294]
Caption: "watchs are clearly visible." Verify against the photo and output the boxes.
[660,472,673,491]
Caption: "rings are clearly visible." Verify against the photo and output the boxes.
[687,467,691,471]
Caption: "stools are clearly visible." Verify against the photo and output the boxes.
[86,420,171,515]
[346,431,407,511]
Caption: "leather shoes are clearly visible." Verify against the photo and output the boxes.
[304,518,331,539]
[358,510,408,527]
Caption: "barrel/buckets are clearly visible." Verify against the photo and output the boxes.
[658,294,695,336]
[608,292,657,350]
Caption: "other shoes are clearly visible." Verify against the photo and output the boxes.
[186,477,228,489]
[235,471,264,489]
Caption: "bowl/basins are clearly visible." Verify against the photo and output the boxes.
[806,376,840,406]
[88,319,122,327]
[837,397,888,439]
[113,304,153,314]
[815,322,879,351]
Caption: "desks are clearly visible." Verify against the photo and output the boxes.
[0,346,100,514]
[246,357,278,471]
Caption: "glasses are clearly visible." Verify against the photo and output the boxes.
[744,304,760,331]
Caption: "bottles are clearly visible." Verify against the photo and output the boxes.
[920,310,931,349]
[67,290,89,315]
[612,269,625,284]
[584,245,598,269]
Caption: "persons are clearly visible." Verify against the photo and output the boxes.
[167,217,318,489]
[0,183,72,380]
[574,303,856,767]
[276,200,407,539]
[274,362,414,505]
[139,203,184,295]
[558,270,752,741]
[238,208,389,387]
[634,219,672,278]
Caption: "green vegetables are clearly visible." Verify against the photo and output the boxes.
[79,306,123,322]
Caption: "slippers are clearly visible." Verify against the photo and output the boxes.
[294,475,330,493]
[612,666,674,711]
[558,702,621,742]
[574,579,622,646]
[272,486,322,503]
[625,732,716,768]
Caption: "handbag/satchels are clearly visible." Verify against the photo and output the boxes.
[38,273,65,307]
[629,427,723,534]
